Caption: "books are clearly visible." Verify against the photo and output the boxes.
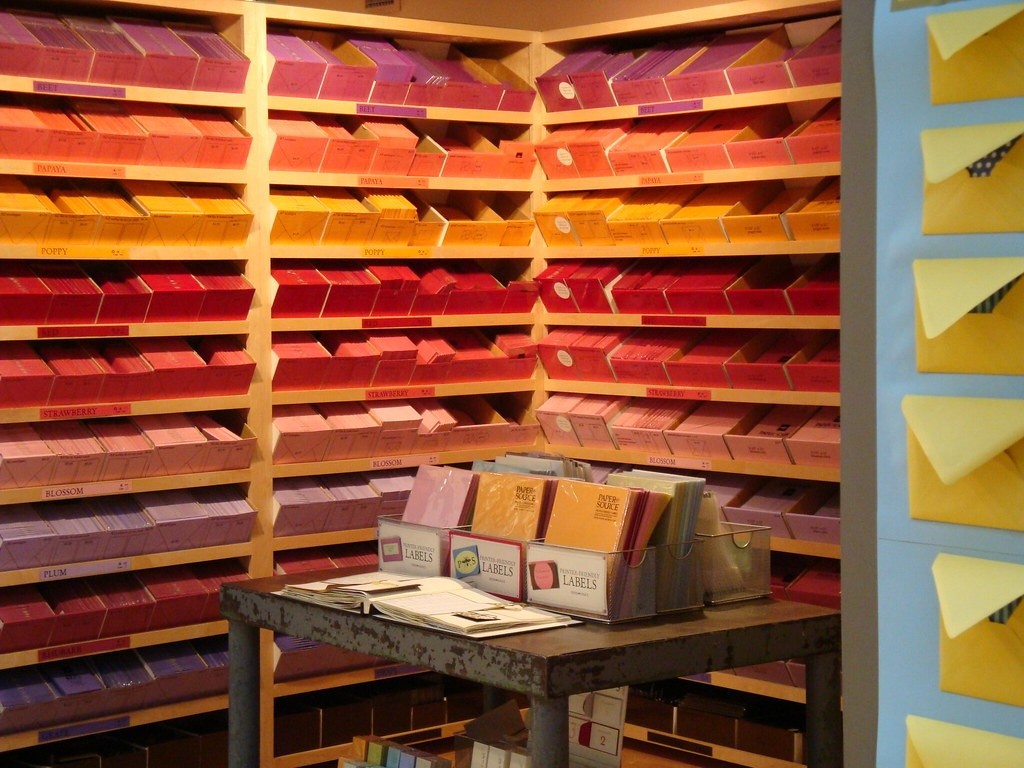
[400,449,747,617]
[270,570,586,641]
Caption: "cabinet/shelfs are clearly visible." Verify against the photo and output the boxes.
[538,0,844,764]
[254,2,536,768]
[0,0,249,754]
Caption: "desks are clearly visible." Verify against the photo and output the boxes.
[218,564,839,768]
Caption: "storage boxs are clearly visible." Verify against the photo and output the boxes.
[0,10,849,768]
